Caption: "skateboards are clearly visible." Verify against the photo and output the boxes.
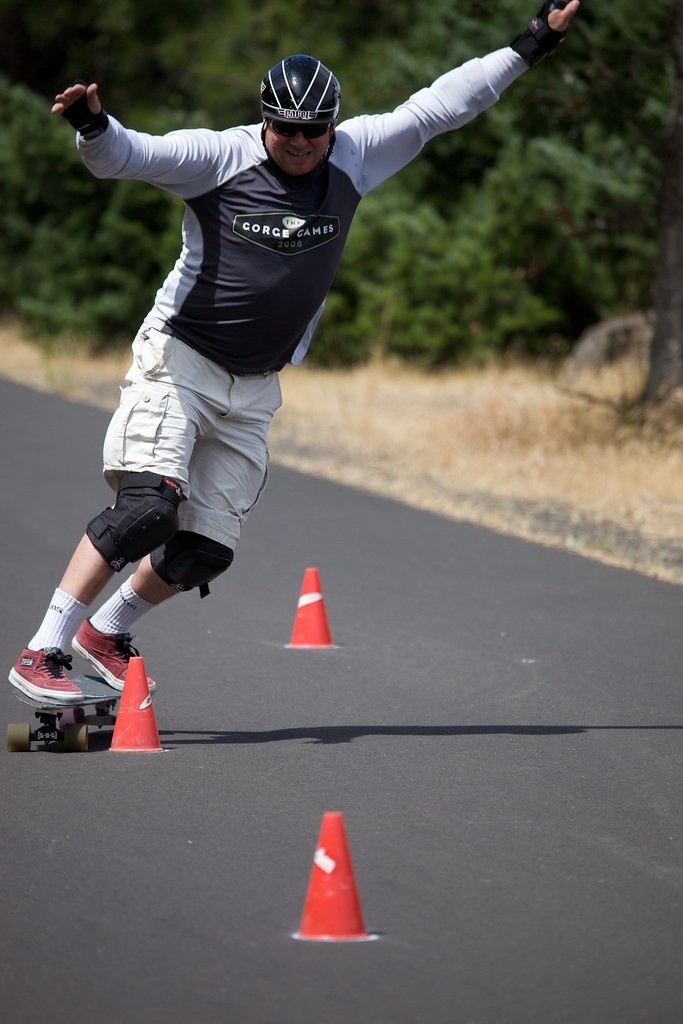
[6,668,158,750]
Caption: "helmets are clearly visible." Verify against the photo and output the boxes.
[260,54,342,125]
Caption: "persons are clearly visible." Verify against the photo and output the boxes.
[7,0,582,706]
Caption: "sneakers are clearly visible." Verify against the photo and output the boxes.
[70,615,157,694]
[7,647,86,705]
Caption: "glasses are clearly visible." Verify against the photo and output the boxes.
[270,119,331,139]
